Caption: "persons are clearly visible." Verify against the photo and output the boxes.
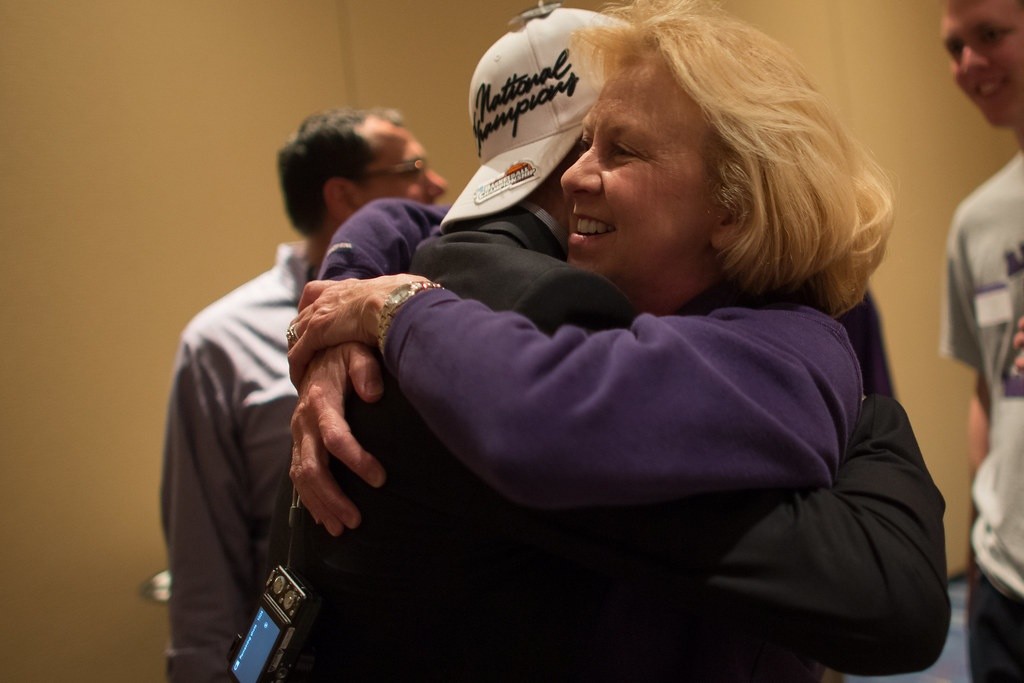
[159,0,1024,683]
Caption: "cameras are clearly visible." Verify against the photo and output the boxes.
[226,562,322,683]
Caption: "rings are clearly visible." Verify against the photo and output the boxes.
[285,325,299,341]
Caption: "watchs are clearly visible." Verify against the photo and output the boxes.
[377,281,444,359]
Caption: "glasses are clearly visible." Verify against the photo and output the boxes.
[355,156,427,181]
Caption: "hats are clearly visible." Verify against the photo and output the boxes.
[440,8,634,235]
